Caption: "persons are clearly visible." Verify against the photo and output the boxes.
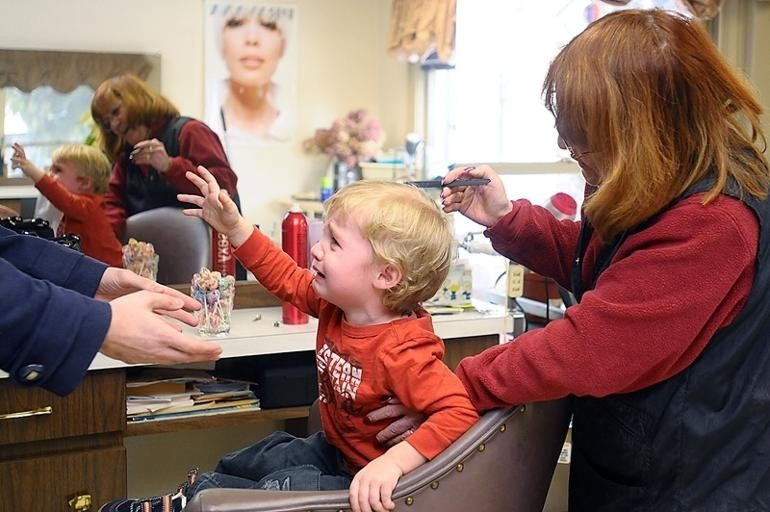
[0,224,223,398]
[209,5,294,142]
[91,73,242,216]
[441,8,770,512]
[10,142,122,268]
[98,165,480,512]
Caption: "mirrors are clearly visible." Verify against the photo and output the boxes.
[0,2,591,308]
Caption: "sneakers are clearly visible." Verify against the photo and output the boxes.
[98,470,197,511]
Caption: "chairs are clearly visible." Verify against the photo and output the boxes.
[178,388,577,512]
[124,207,214,284]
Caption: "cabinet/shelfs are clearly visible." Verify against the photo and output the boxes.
[1,299,516,512]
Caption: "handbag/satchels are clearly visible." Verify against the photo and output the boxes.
[0,216,55,238]
[21,231,82,253]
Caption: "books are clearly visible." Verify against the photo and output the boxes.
[126,374,262,423]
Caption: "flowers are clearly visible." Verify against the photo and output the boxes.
[301,106,387,166]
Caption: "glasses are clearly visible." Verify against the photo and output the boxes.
[102,100,121,132]
[547,91,594,163]
